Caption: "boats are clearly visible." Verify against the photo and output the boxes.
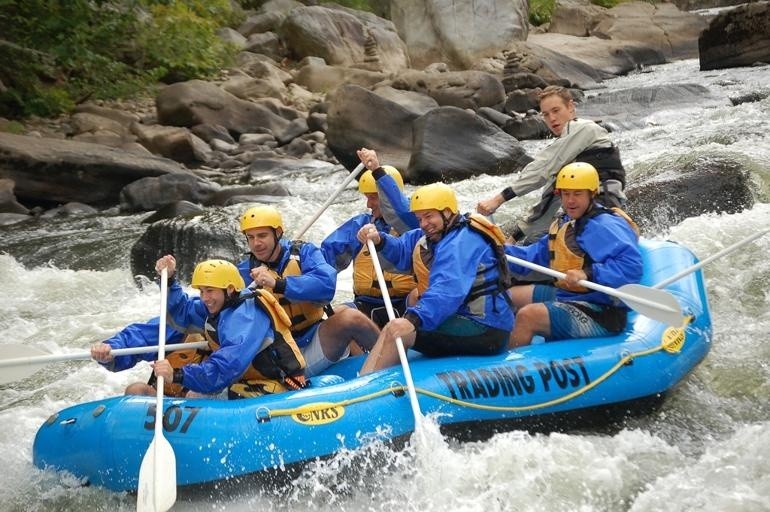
[31,240,716,492]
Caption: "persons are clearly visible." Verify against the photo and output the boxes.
[506,161,644,350]
[234,205,381,379]
[90,294,217,400]
[358,182,516,378]
[320,146,421,353]
[474,85,626,248]
[149,254,312,399]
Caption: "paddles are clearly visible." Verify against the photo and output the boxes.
[504,254,683,327]
[367,237,444,481]
[137,267,176,512]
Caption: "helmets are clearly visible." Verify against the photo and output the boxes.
[556,162,600,194]
[240,206,283,232]
[192,259,245,292]
[358,165,404,192]
[409,182,458,215]
